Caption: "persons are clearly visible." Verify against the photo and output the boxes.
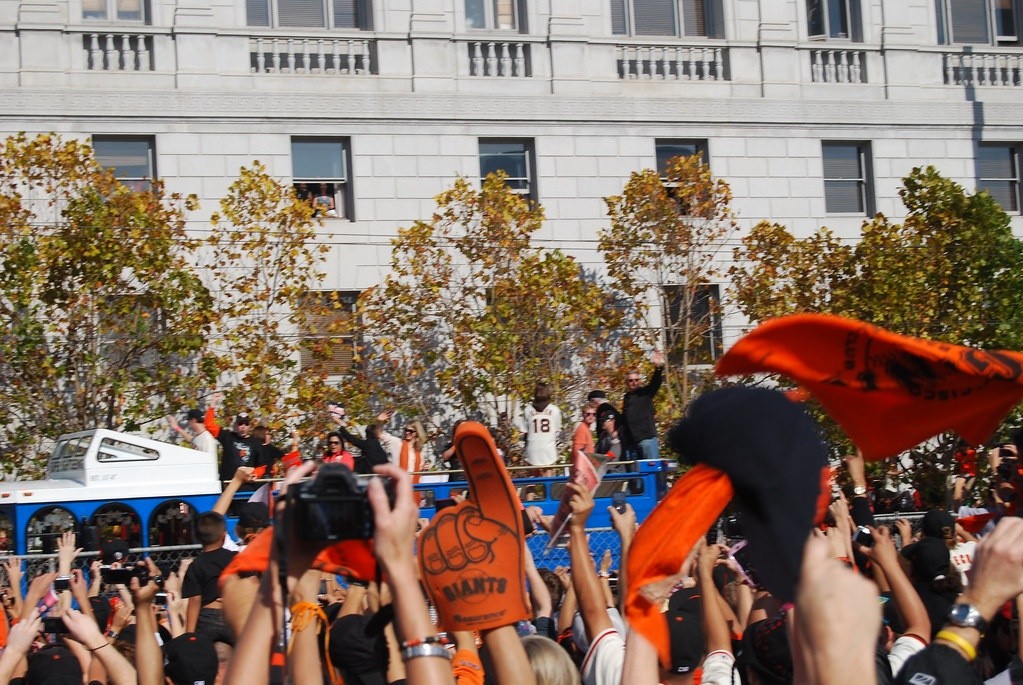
[0,357,1023,684]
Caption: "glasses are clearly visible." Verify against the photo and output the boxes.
[599,412,616,423]
[627,379,639,382]
[238,422,249,427]
[404,427,417,435]
[587,413,596,416]
[328,441,339,445]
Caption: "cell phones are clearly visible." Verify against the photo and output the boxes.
[613,492,626,515]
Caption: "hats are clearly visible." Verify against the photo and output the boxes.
[739,614,793,685]
[160,632,219,685]
[29,647,83,685]
[328,605,395,685]
[90,595,112,633]
[919,510,956,538]
[185,409,204,419]
[903,539,951,585]
[660,586,707,673]
[237,412,250,422]
[238,502,272,529]
[99,538,129,562]
[712,563,736,592]
[587,390,606,401]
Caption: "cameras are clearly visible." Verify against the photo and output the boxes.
[104,566,149,587]
[40,617,72,634]
[54,575,77,590]
[852,525,876,548]
[282,462,398,544]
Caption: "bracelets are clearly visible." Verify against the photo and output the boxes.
[90,641,110,651]
[935,630,978,660]
[325,579,333,582]
[289,603,336,683]
[399,636,450,648]
[403,644,451,660]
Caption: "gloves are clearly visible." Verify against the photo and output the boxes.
[418,419,533,633]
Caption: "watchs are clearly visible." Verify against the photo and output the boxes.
[947,603,990,634]
[853,486,868,495]
